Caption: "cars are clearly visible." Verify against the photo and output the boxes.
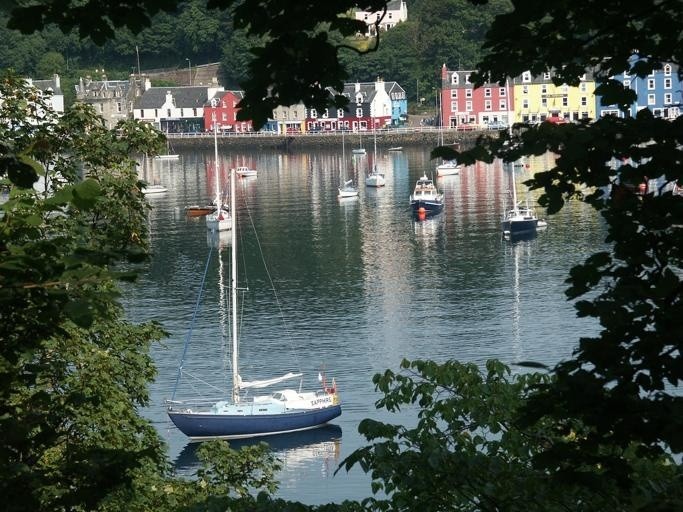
[225,130,240,135]
[336,127,353,133]
[310,127,323,134]
[285,127,301,135]
[456,122,476,130]
[261,129,276,135]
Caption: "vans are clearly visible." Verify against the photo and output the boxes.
[544,116,570,125]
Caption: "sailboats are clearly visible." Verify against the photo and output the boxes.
[502,79,547,233]
[167,424,342,486]
[205,120,233,231]
[153,122,180,157]
[206,229,232,360]
[167,168,341,440]
[364,125,387,186]
[436,174,460,200]
[435,92,461,175]
[503,235,544,364]
[366,186,387,198]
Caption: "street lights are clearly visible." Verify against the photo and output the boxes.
[185,57,192,86]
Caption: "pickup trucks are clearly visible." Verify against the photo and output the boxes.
[488,119,510,130]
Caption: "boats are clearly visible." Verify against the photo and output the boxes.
[143,192,166,200]
[404,169,446,210]
[234,167,257,176]
[354,154,365,157]
[141,185,167,194]
[389,151,402,155]
[388,146,402,151]
[671,183,682,197]
[337,197,358,205]
[352,148,365,154]
[337,180,358,197]
[408,210,444,248]
